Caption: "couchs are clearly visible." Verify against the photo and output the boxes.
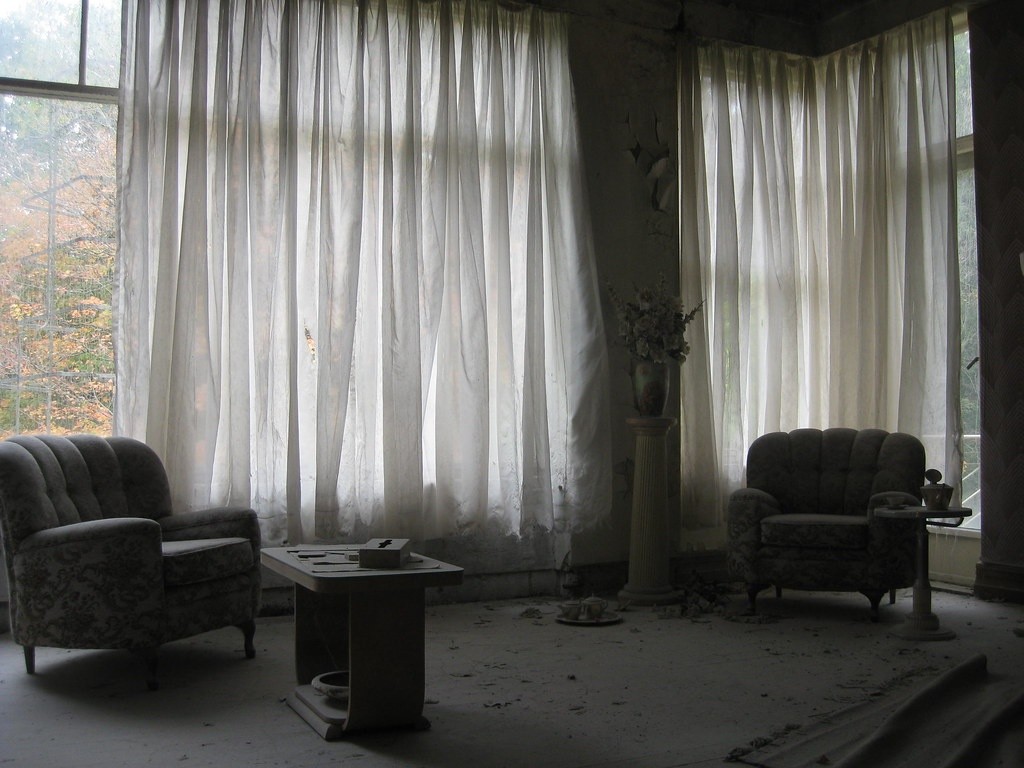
[1,431,262,695]
[726,427,927,617]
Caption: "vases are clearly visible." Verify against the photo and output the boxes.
[630,352,671,416]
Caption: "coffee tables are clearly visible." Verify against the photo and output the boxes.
[260,543,465,742]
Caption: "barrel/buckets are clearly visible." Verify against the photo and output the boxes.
[920,487,954,510]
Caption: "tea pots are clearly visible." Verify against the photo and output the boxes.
[558,598,584,620]
[583,594,608,618]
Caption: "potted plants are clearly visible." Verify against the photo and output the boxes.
[920,468,954,510]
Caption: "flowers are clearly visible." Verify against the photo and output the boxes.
[604,269,709,368]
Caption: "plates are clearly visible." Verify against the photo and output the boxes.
[885,504,907,509]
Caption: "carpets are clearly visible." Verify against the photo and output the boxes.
[724,651,1024,768]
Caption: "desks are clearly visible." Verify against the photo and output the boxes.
[874,504,972,643]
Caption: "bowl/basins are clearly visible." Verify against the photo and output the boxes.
[311,670,349,701]
[888,497,904,504]
